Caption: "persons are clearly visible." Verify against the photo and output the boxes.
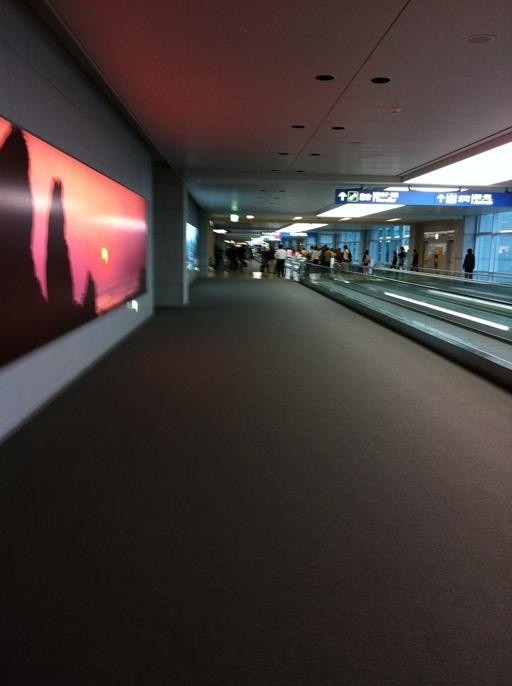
[410,248,418,272]
[390,250,399,269]
[397,247,406,270]
[362,249,371,275]
[213,242,353,278]
[461,247,475,286]
